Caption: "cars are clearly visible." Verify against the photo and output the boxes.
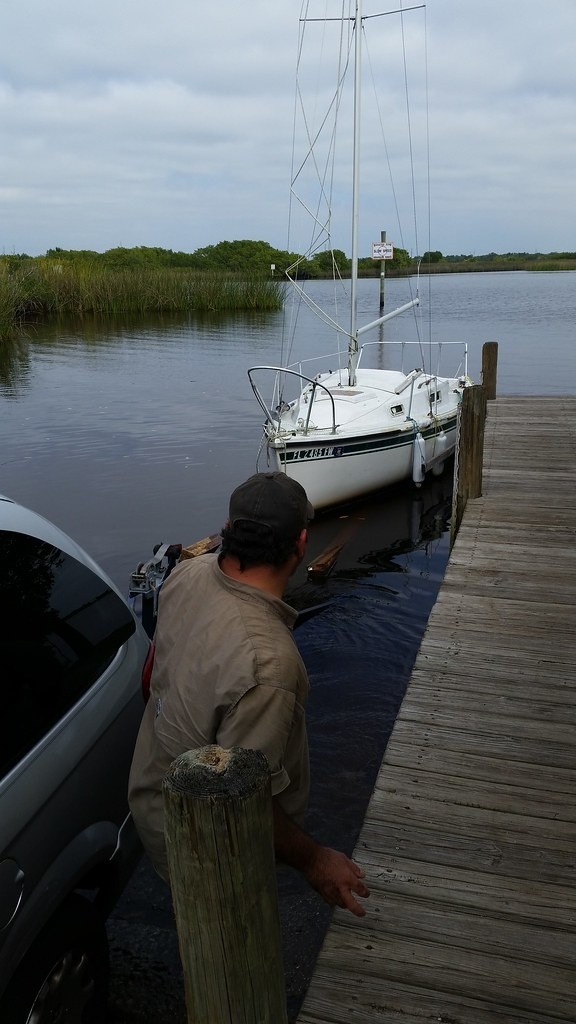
[0,491,157,1023]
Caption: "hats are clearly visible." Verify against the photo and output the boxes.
[229,471,314,543]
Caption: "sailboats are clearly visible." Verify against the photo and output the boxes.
[245,0,475,510]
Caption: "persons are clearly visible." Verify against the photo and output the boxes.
[124,470,372,918]
